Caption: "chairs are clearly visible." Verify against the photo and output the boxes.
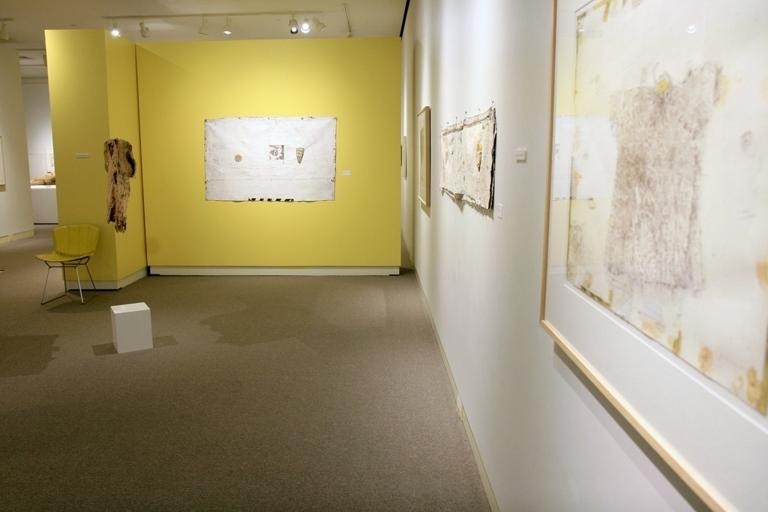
[33,224,98,305]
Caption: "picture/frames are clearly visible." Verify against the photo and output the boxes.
[415,105,431,210]
[537,0,768,512]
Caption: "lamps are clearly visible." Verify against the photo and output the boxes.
[288,11,326,35]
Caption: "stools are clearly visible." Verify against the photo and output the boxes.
[109,302,154,355]
